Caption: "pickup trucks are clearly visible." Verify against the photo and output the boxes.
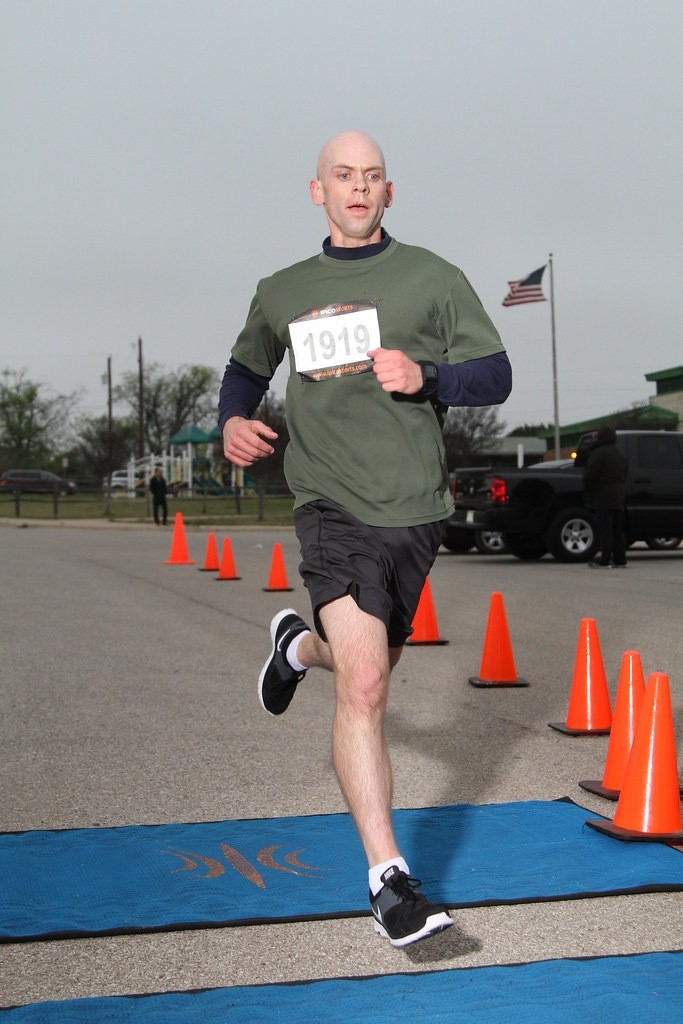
[450,429,683,563]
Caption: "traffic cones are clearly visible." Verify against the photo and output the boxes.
[546,615,612,736]
[468,593,531,687]
[164,512,195,564]
[577,649,646,799]
[262,543,295,592]
[584,672,682,845]
[404,576,450,646]
[213,537,242,581]
[197,532,220,571]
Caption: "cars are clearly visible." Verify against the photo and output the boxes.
[442,459,577,555]
[102,470,140,492]
[0,470,77,497]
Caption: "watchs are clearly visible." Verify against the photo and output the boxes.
[416,361,439,396]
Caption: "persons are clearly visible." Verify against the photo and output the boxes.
[583,427,629,568]
[150,467,169,526]
[218,128,513,950]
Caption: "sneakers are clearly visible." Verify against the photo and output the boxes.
[369,866,454,946]
[258,608,309,717]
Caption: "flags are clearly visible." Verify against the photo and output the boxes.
[502,264,549,307]
